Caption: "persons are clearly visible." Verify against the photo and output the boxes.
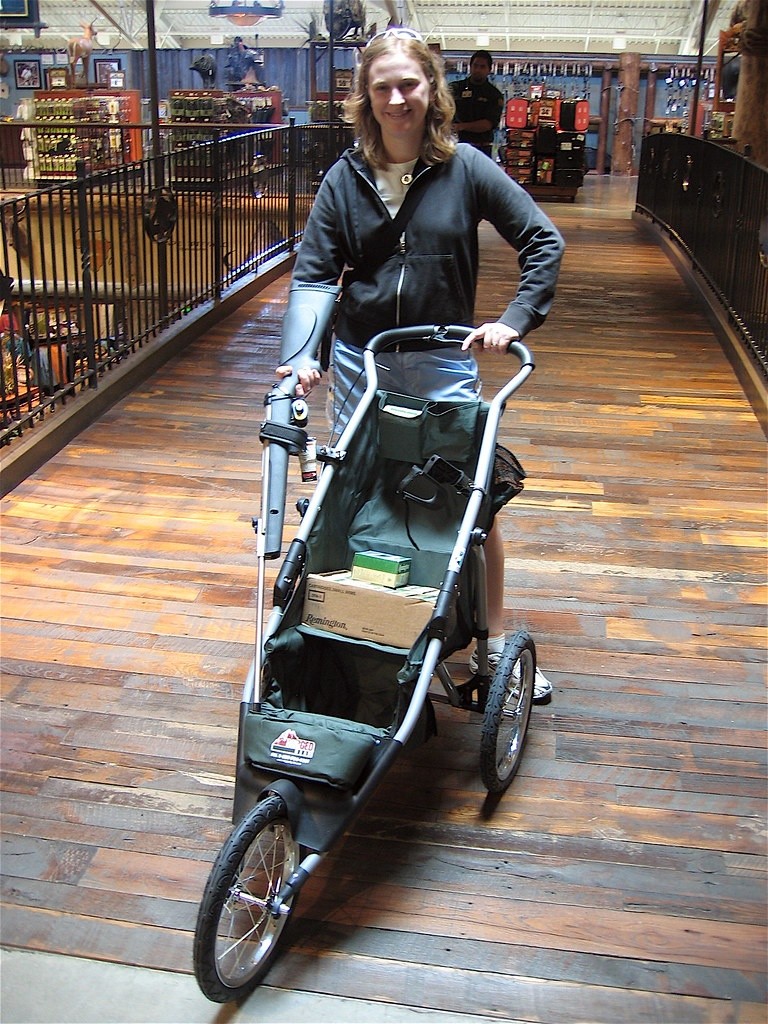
[275,30,566,702]
[18,63,35,86]
[447,50,504,159]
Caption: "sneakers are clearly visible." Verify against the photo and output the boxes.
[469,648,553,699]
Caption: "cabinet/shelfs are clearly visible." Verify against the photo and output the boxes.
[169,88,289,188]
[34,89,145,189]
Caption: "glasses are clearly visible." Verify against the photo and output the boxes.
[365,27,425,50]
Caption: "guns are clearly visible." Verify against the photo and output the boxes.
[252,277,346,709]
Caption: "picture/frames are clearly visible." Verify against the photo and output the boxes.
[43,69,50,90]
[13,59,42,90]
[94,58,121,84]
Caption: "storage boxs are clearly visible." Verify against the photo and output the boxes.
[506,96,590,187]
[303,569,440,648]
[352,550,412,588]
[170,96,214,165]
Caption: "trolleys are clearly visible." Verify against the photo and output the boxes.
[186,322,542,1007]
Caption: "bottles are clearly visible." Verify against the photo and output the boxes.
[34,98,101,171]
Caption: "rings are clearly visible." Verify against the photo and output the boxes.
[483,346,490,350]
[491,341,498,348]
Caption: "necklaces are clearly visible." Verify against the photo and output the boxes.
[385,153,418,185]
